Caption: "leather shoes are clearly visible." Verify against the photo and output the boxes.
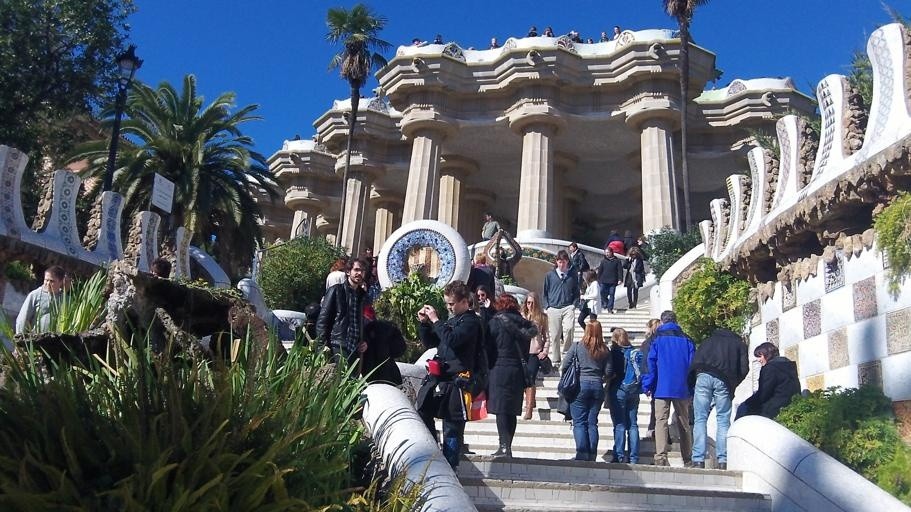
[491,446,511,457]
[688,462,704,467]
[719,462,726,469]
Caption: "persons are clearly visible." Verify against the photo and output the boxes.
[642,310,699,468]
[561,319,611,460]
[481,211,500,241]
[543,250,580,368]
[413,39,423,44]
[526,26,621,43]
[150,258,173,279]
[299,253,550,475]
[490,38,498,50]
[603,329,644,465]
[733,342,802,422]
[15,265,68,333]
[569,229,652,329]
[684,329,749,469]
[639,318,673,445]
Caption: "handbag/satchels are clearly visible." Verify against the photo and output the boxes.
[558,358,580,400]
[579,299,586,310]
[521,358,529,387]
[538,357,551,374]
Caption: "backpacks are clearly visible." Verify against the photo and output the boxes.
[610,346,644,393]
[455,314,497,396]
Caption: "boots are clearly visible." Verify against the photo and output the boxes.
[523,387,535,418]
